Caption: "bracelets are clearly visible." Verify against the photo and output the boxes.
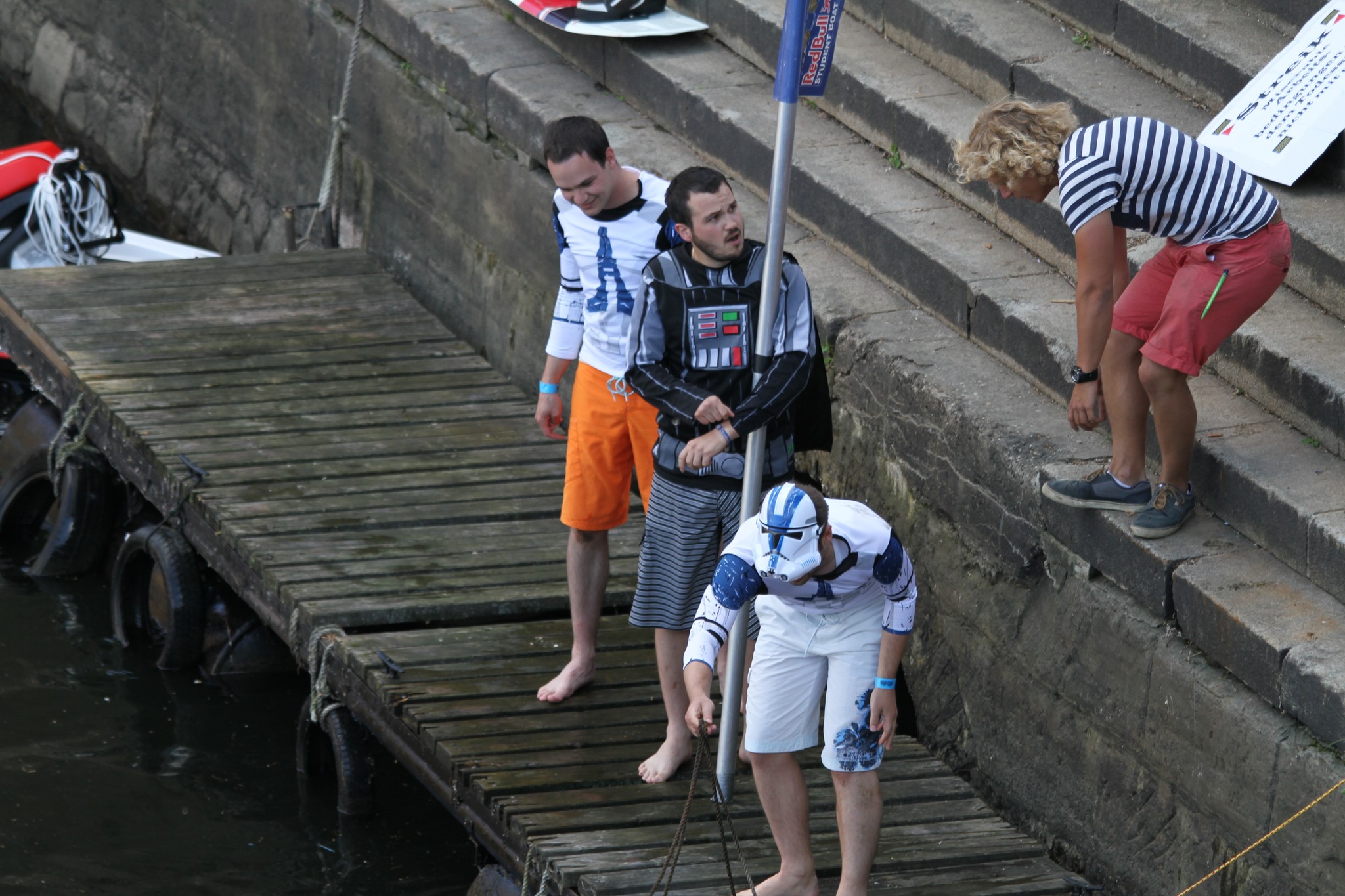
[874,676,896,689]
[538,379,561,394]
[716,423,732,447]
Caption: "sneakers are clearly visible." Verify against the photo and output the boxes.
[1042,464,1152,513]
[1131,481,1197,538]
[575,0,667,22]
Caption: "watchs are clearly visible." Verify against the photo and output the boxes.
[1071,365,1099,383]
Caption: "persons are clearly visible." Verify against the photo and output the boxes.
[951,97,1292,538]
[682,477,918,896]
[624,164,820,784]
[532,116,753,715]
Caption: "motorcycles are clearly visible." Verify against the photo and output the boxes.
[0,141,225,380]
